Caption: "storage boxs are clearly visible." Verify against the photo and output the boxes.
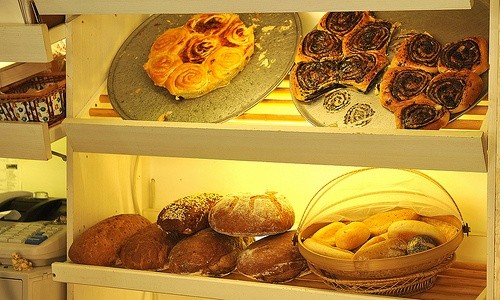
[0,72,66,126]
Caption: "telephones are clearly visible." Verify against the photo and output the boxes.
[0,190,67,266]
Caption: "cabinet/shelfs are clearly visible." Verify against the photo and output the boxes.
[0,0,500,300]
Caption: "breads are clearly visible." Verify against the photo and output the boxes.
[376,31,489,130]
[303,208,448,262]
[290,10,401,101]
[144,14,255,99]
[68,192,309,283]
